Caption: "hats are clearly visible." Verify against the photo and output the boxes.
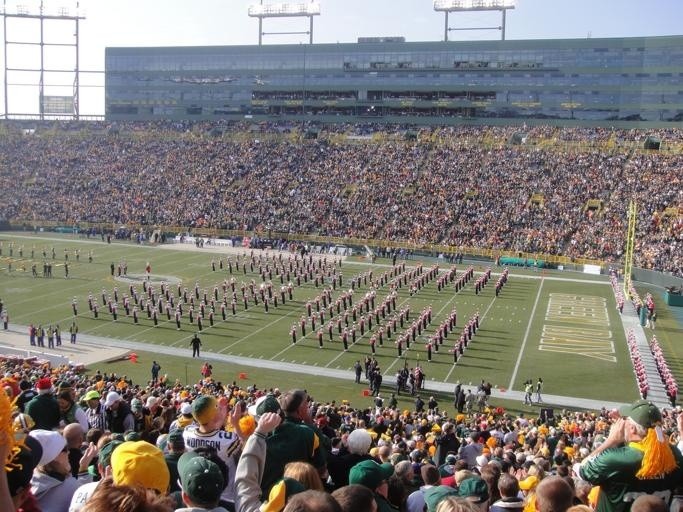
[618,399,662,429]
[0,376,610,504]
